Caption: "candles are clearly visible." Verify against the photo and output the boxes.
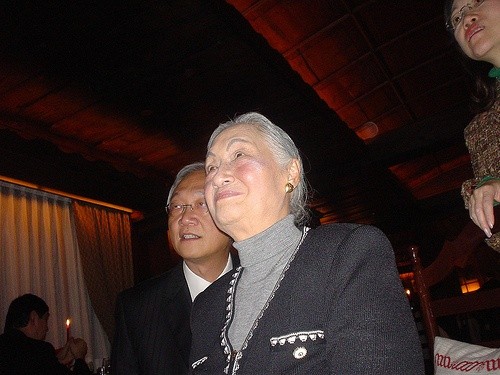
[67,323,70,342]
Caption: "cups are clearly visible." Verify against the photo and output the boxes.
[102,358,111,375]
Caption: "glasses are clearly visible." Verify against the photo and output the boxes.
[164,198,210,217]
[445,0,486,31]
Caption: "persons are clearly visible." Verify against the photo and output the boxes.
[443,0,500,252]
[0,294,93,375]
[110,161,241,375]
[186,111,424,375]
